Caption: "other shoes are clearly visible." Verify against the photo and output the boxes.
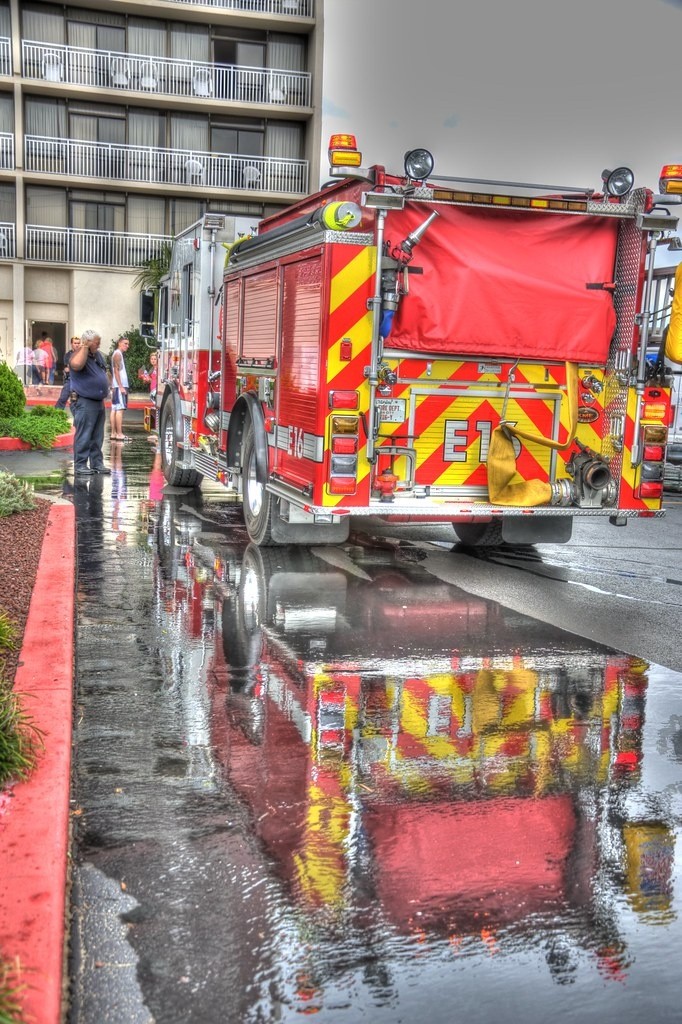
[110,435,117,440]
[116,434,132,440]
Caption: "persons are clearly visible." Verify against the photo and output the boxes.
[147,439,168,519]
[72,476,105,602]
[16,331,58,385]
[55,336,81,410]
[109,442,129,541]
[69,329,111,476]
[143,352,157,406]
[110,337,132,441]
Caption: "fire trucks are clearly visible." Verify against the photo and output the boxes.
[134,132,681,547]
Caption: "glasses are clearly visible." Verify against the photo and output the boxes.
[95,358,104,368]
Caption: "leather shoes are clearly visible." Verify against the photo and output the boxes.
[74,468,94,475]
[92,466,111,473]
